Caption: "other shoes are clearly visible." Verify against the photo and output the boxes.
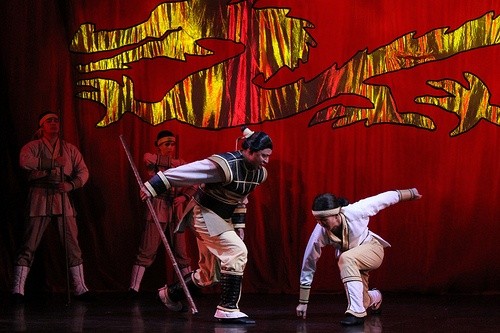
[373,288,383,315]
[9,293,24,305]
[128,291,137,299]
[340,315,364,325]
[74,291,101,301]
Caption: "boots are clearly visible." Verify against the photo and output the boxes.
[214,269,248,318]
[158,272,204,311]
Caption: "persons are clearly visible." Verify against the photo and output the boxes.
[296,188,421,326]
[140,126,273,323]
[127,130,192,303]
[10,113,100,301]
